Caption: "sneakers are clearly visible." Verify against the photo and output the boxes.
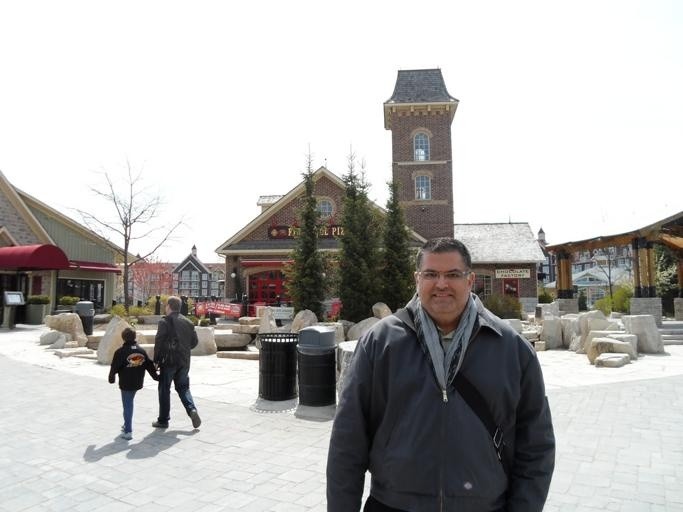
[152,422,168,427]
[120,425,131,440]
[190,409,201,428]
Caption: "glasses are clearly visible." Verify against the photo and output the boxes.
[419,271,471,280]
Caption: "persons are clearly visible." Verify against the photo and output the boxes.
[151,296,201,428]
[327,236,555,512]
[108,328,158,440]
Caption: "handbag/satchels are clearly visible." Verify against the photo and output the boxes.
[158,352,182,373]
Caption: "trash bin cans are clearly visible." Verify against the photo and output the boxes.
[296,325,338,407]
[256,333,299,401]
[75,301,95,335]
